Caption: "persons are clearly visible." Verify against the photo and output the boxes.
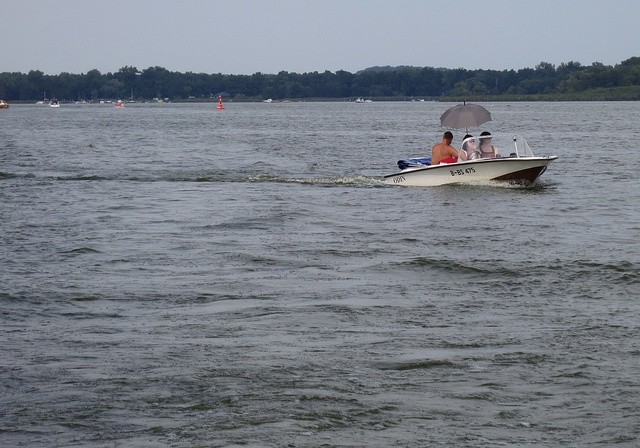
[457,135,483,163]
[431,130,459,164]
[478,132,498,158]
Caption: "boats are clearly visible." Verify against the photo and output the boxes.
[0,100,9,108]
[49,98,60,107]
[381,133,559,187]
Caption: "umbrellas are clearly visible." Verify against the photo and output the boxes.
[439,101,492,134]
